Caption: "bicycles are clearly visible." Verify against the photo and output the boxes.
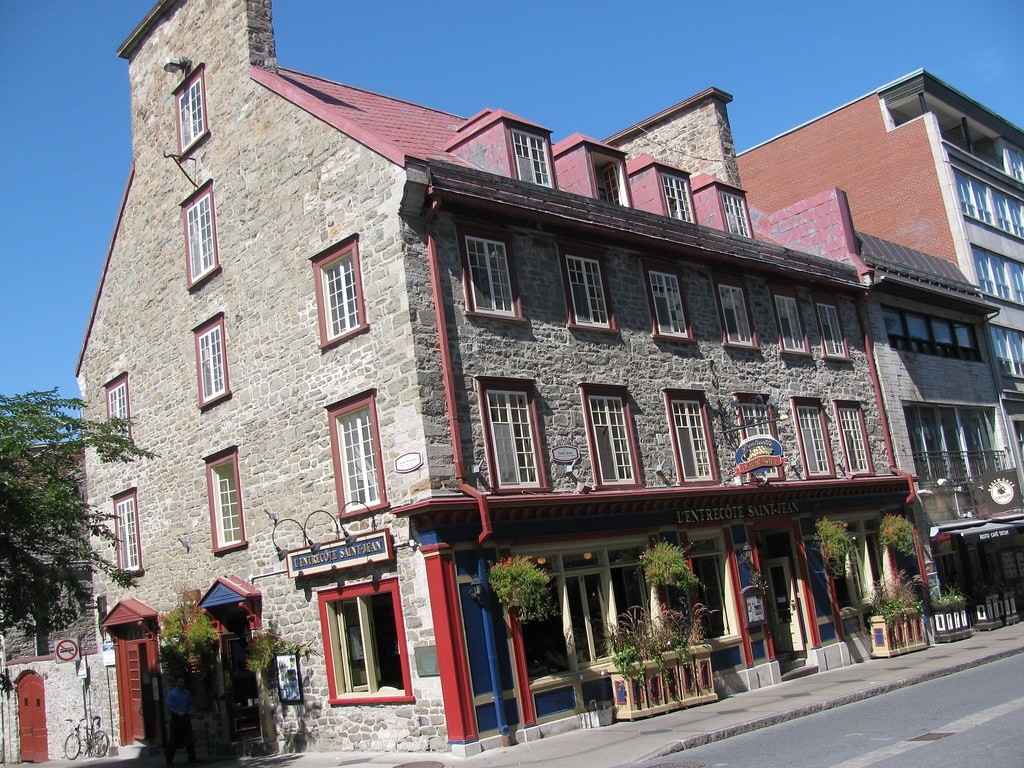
[63,715,109,760]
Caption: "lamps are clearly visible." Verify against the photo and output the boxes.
[469,571,484,602]
[162,56,193,74]
[272,519,308,561]
[303,510,340,555]
[810,533,822,554]
[741,541,753,559]
[339,500,377,548]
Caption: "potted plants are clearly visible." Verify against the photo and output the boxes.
[602,599,720,722]
[485,552,565,623]
[632,535,709,598]
[929,583,973,644]
[862,567,930,658]
[966,572,1021,632]
